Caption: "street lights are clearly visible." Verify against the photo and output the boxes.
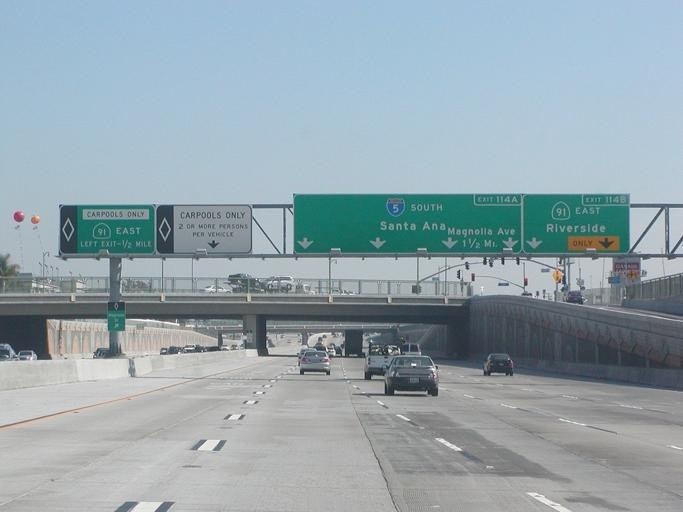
[37,251,73,293]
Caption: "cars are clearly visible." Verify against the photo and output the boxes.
[92,347,115,359]
[0,342,38,361]
[158,342,244,354]
[563,289,585,305]
[200,273,358,298]
[381,355,441,397]
[295,331,344,375]
[482,352,514,377]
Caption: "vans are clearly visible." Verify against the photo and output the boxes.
[399,343,422,357]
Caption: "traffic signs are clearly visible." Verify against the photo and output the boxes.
[58,203,152,258]
[497,283,509,286]
[294,193,522,256]
[519,194,630,256]
[107,301,126,331]
[154,204,252,256]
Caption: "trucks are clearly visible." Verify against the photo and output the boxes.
[363,338,401,379]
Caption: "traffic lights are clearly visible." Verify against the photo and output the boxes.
[464,262,469,270]
[515,257,519,266]
[418,284,421,295]
[524,277,527,286]
[456,269,460,279]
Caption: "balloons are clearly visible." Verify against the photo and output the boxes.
[13,211,24,222]
[30,215,39,224]
